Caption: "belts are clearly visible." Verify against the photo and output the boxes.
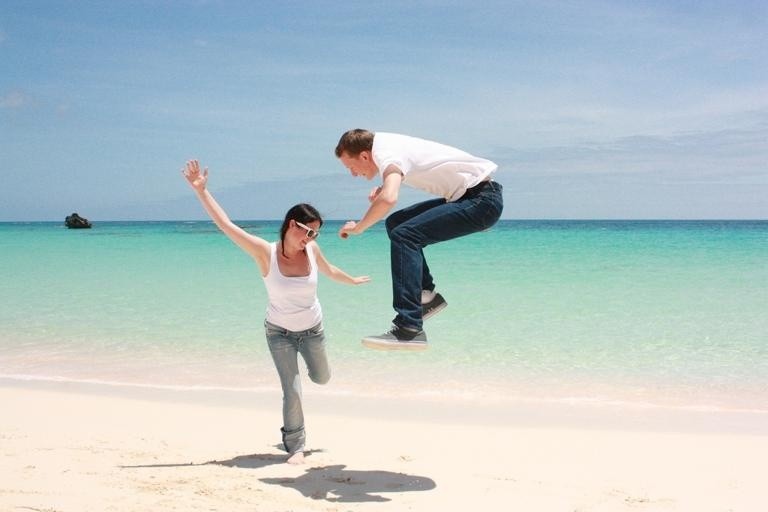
[480,181,502,190]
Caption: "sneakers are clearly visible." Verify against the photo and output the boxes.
[362,327,427,351]
[422,293,448,320]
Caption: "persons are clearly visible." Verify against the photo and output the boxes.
[333,129,505,349]
[180,159,370,465]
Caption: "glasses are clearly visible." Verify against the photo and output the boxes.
[295,221,319,240]
